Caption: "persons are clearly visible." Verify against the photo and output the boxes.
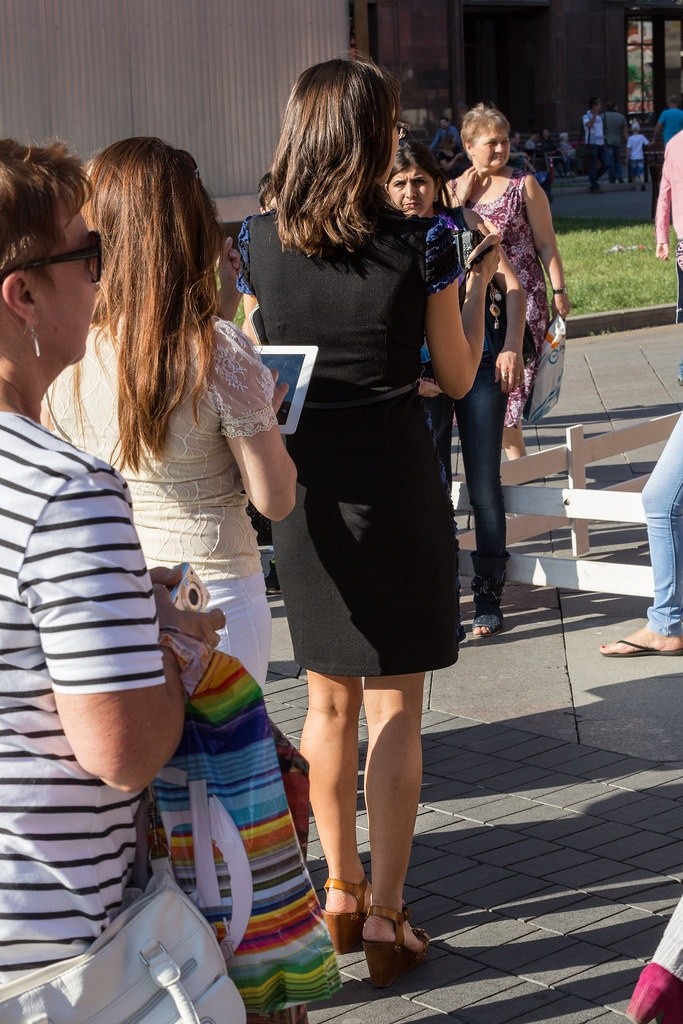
[428,117,465,178]
[583,96,608,193]
[441,102,570,461]
[383,141,527,636]
[599,101,629,183]
[509,128,585,177]
[626,121,652,191]
[652,95,683,145]
[602,406,683,657]
[655,129,683,272]
[0,137,228,984]
[235,59,500,986]
[40,135,345,1024]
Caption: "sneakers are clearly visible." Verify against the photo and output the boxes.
[262,558,283,594]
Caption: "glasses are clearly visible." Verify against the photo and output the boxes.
[391,120,410,140]
[173,150,199,181]
[0,231,102,283]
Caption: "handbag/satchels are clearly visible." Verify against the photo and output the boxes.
[151,623,342,1014]
[1,868,247,1024]
[576,143,593,160]
[523,314,566,422]
[248,719,310,1024]
[449,207,538,366]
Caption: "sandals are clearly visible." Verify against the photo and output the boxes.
[471,601,504,637]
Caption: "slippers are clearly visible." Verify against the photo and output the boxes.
[600,640,683,657]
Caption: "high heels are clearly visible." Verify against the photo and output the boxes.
[325,877,377,953]
[365,904,431,988]
[626,963,683,1024]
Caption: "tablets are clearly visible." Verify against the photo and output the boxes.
[256,345,319,434]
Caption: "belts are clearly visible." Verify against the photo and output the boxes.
[303,380,419,409]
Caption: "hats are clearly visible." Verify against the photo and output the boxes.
[631,122,640,131]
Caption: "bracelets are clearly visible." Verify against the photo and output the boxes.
[553,288,566,294]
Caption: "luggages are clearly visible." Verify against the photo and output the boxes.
[530,144,553,203]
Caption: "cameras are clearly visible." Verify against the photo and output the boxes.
[167,563,212,614]
